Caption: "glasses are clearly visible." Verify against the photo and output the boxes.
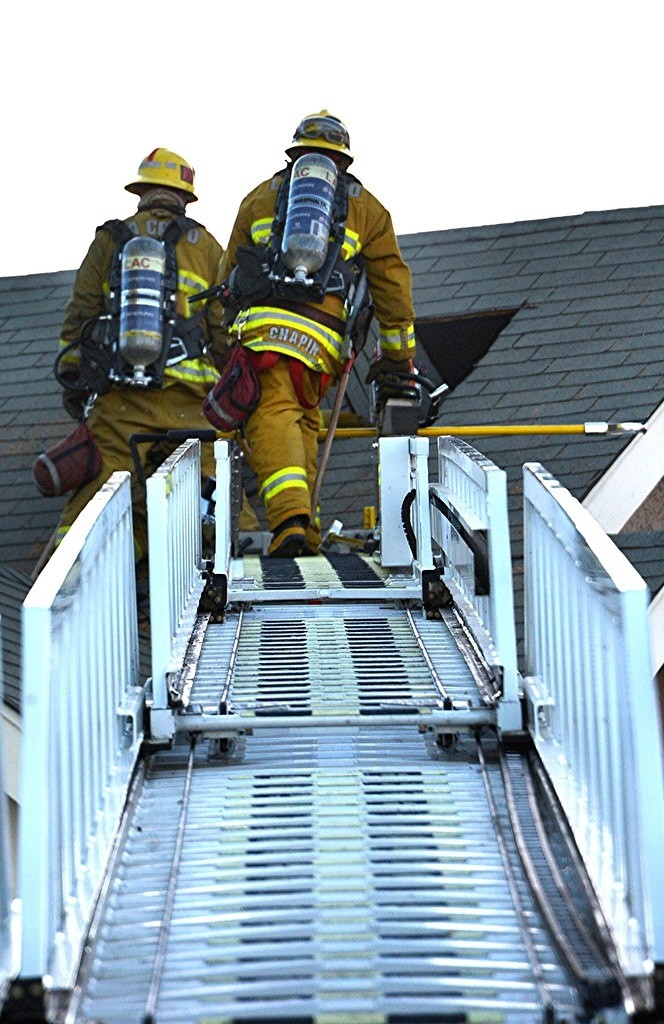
[294,118,349,149]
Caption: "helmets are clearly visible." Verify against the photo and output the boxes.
[124,147,198,202]
[284,109,354,164]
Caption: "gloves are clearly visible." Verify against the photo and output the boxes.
[63,389,88,423]
[364,358,415,388]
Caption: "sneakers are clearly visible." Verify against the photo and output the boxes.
[267,515,305,557]
[305,526,322,556]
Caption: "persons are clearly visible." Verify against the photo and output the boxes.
[53,148,225,562]
[218,109,417,556]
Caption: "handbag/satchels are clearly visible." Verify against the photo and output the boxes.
[202,339,261,431]
[33,423,102,497]
